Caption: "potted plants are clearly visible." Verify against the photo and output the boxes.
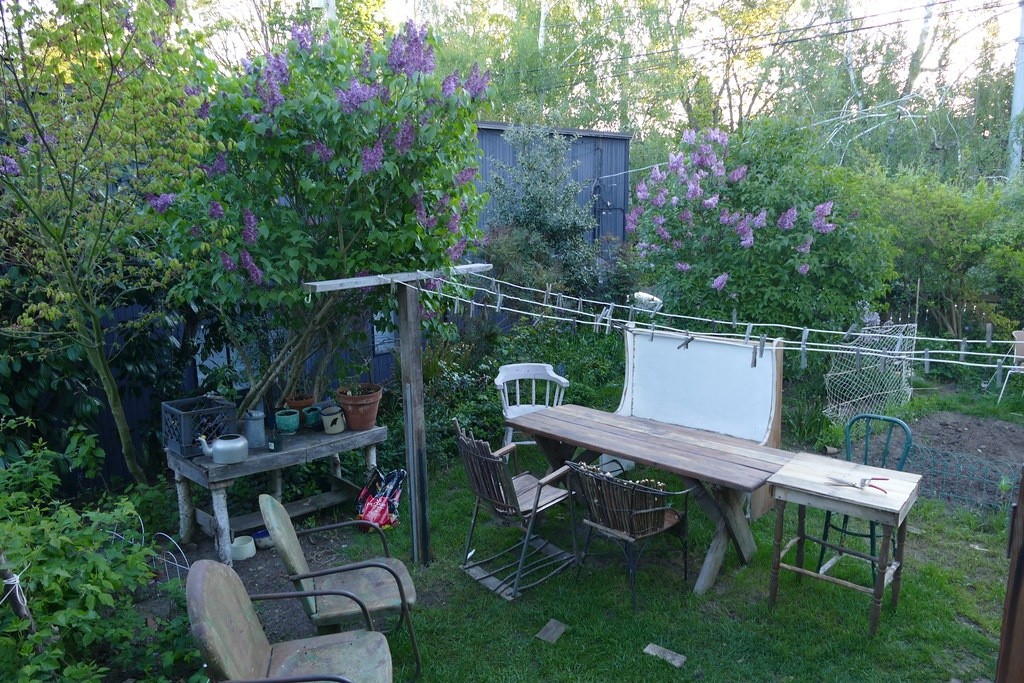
[286,377,315,422]
[336,375,383,432]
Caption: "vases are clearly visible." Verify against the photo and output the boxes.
[275,410,299,435]
[302,406,321,427]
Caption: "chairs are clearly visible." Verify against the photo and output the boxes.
[451,417,581,605]
[187,559,393,683]
[815,414,913,592]
[566,459,702,616]
[258,493,422,683]
[997,331,1024,409]
[495,362,570,465]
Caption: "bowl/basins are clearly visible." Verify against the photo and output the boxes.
[229,529,275,561]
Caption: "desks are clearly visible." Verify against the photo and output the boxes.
[505,403,798,597]
[768,451,922,640]
[165,420,388,570]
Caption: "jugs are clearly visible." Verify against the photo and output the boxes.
[195,432,249,465]
[243,409,266,448]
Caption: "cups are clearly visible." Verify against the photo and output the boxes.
[319,405,346,434]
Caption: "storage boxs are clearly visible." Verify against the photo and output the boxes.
[160,396,236,458]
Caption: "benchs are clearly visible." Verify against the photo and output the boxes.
[598,323,786,526]
